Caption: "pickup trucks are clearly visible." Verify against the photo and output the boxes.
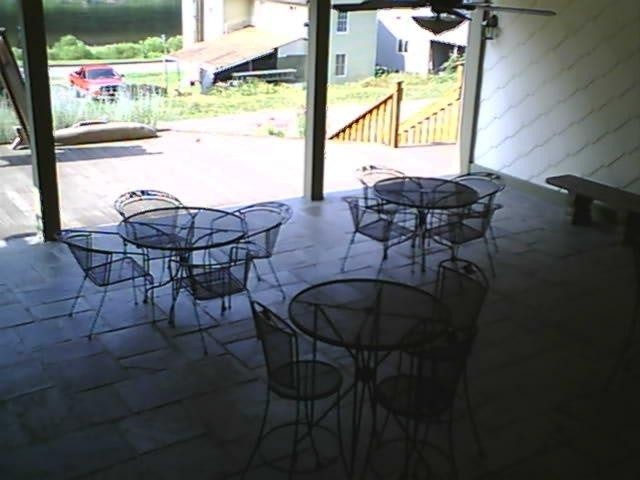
[69,64,126,103]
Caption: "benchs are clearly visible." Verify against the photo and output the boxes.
[546,174,640,246]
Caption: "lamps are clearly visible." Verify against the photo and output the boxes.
[481,15,498,40]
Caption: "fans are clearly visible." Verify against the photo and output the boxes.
[331,0,557,35]
